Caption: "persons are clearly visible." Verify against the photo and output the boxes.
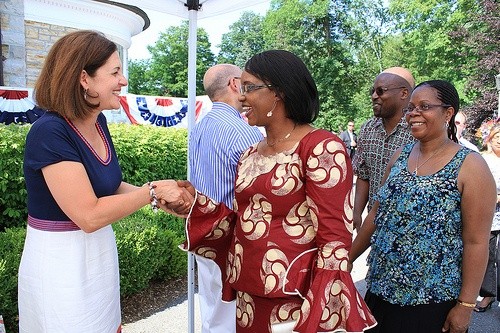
[161,49,378,333]
[455,110,500,312]
[350,79,497,333]
[340,120,357,159]
[189,63,265,333]
[18,30,193,333]
[354,66,418,231]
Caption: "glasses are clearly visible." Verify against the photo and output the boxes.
[240,84,273,95]
[402,105,452,114]
[372,87,406,96]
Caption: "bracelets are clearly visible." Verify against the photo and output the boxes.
[456,299,476,309]
[146,181,158,213]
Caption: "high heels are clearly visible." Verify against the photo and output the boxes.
[474,297,495,312]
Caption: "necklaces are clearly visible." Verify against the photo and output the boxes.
[413,141,450,174]
[266,119,296,147]
